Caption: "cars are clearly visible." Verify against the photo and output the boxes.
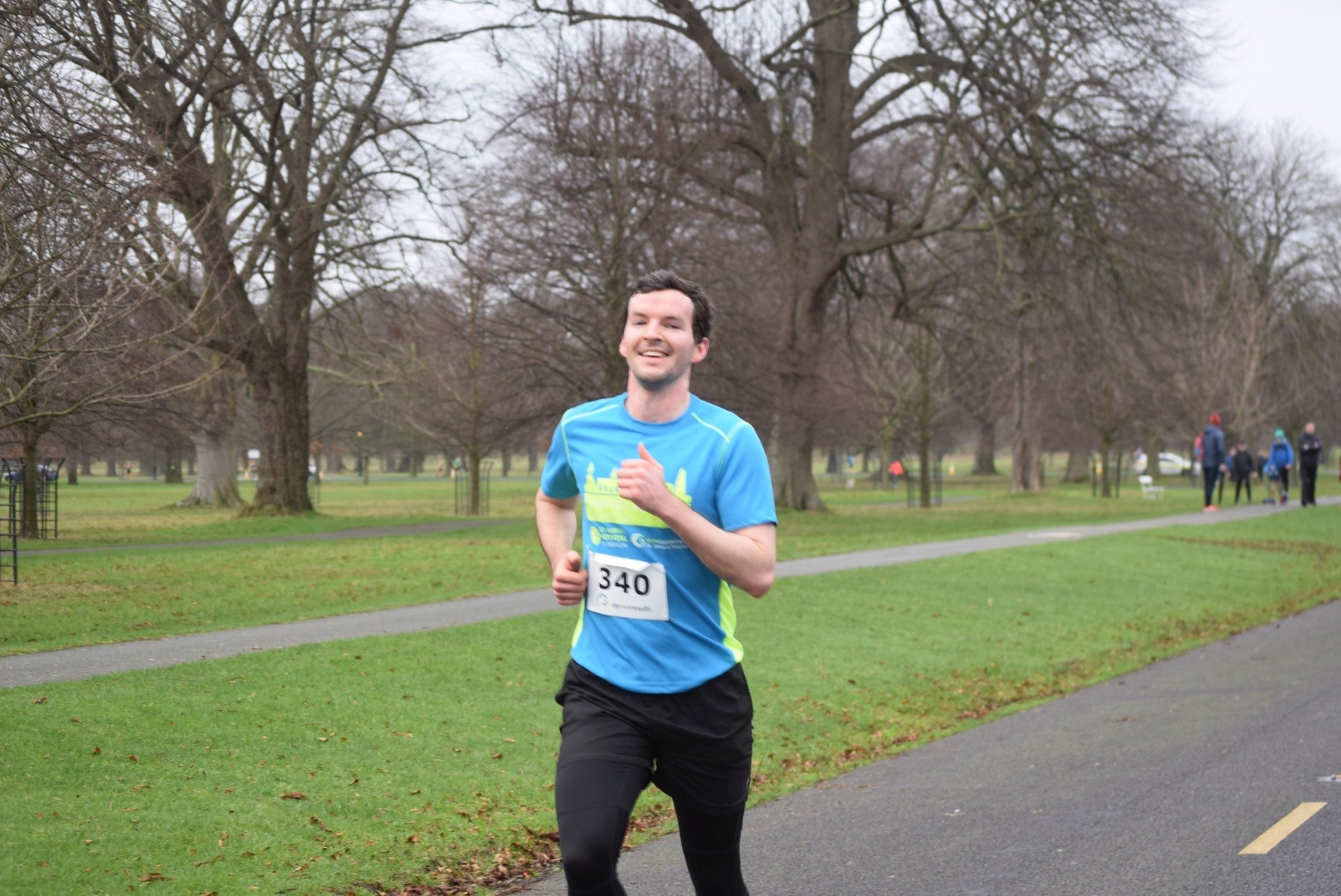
[1131,452,1201,478]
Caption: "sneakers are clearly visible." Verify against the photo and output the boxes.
[1205,505,1218,511]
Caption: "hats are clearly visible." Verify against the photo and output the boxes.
[1210,413,1221,425]
[1274,428,1285,439]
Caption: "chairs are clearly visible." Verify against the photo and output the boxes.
[1138,474,1164,500]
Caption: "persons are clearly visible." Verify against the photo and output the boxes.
[1225,429,1293,504]
[1298,423,1322,507]
[1200,413,1225,512]
[535,271,778,896]
